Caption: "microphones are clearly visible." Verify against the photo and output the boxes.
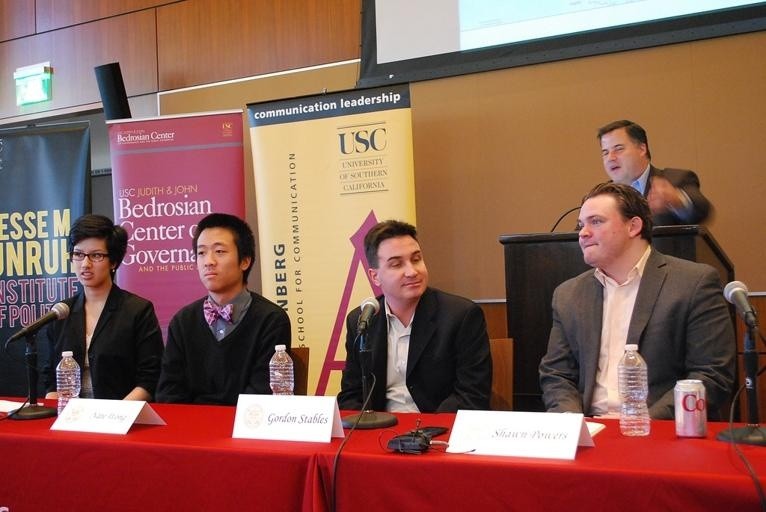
[7,302,70,346]
[360,298,380,331]
[551,177,614,233]
[723,282,760,328]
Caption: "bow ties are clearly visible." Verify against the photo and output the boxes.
[203,300,233,325]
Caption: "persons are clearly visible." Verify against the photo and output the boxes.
[337,219,494,413]
[595,118,713,263]
[156,216,292,407]
[537,181,740,421]
[34,214,164,402]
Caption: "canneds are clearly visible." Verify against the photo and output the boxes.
[674,379,707,437]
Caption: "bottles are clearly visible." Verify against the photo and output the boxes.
[619,344,650,436]
[269,345,295,394]
[56,348,81,416]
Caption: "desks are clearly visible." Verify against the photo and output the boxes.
[1,394,764,512]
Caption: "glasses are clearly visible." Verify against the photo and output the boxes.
[69,251,110,262]
